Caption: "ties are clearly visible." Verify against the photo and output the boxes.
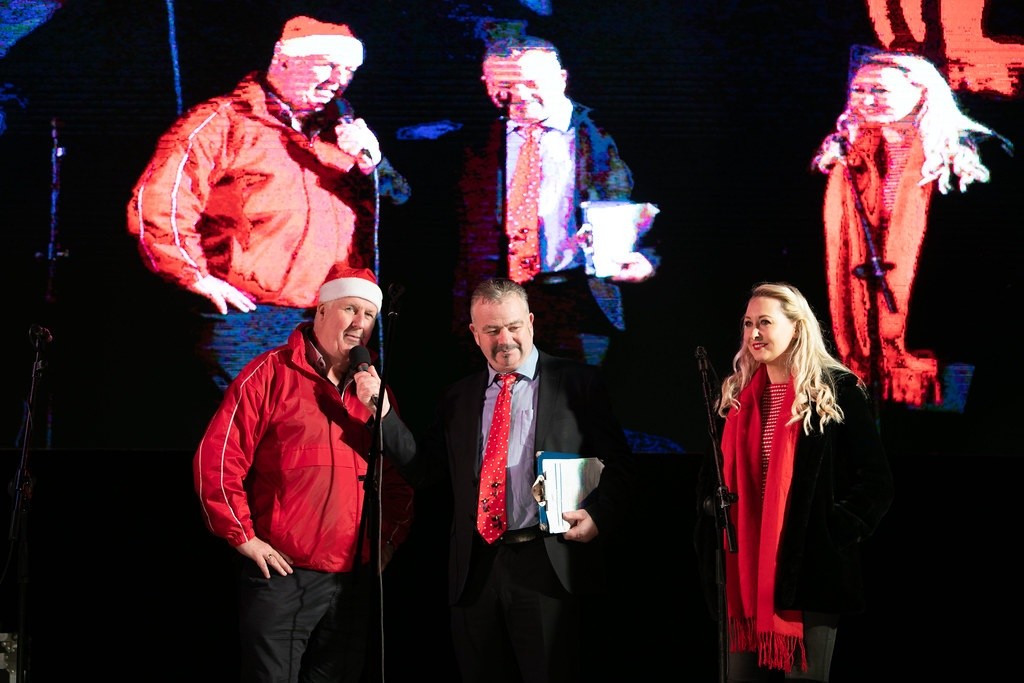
[477,373,521,544]
[506,122,548,285]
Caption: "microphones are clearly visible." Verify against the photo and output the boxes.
[52,119,67,158]
[329,98,373,159]
[31,324,52,342]
[695,346,721,394]
[832,130,850,143]
[349,344,379,407]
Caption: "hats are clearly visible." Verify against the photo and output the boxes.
[271,15,363,67]
[319,257,383,314]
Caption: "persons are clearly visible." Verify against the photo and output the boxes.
[354,277,635,683]
[694,283,894,683]
[807,51,1024,417]
[127,16,378,411]
[195,261,416,683]
[334,35,689,361]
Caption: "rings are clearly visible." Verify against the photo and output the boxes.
[268,554,272,560]
